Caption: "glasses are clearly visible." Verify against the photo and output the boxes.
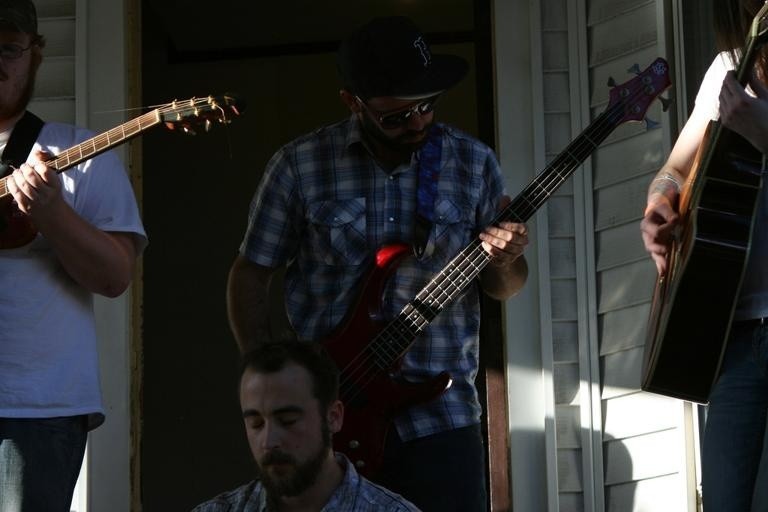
[2,36,40,59]
[353,95,438,135]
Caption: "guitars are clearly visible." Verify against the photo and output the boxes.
[314,58,672,470]
[0,95,239,250]
[640,0,767,404]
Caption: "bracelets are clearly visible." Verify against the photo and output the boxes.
[648,174,681,192]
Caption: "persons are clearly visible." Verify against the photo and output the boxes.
[638,0,766,511]
[188,331,425,511]
[224,30,534,511]
[0,1,152,511]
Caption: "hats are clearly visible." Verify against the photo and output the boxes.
[345,11,471,100]
